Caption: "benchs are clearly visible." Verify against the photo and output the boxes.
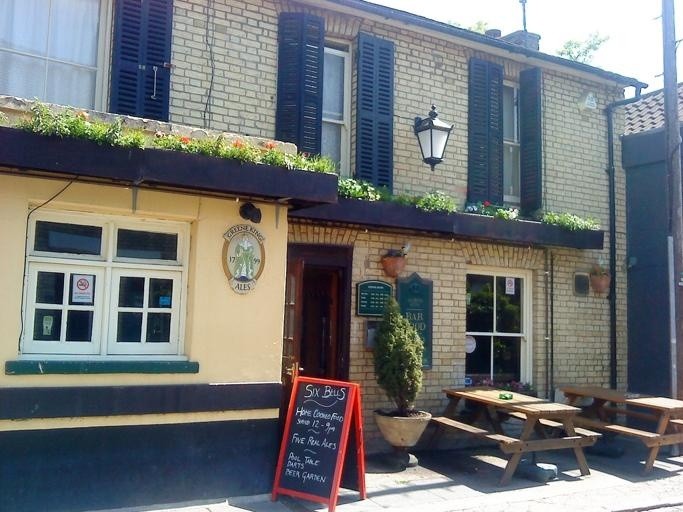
[428,406,683,486]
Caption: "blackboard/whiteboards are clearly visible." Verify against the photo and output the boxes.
[274,376,356,503]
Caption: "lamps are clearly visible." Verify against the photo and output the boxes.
[412,104,456,172]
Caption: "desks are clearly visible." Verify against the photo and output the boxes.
[441,385,683,487]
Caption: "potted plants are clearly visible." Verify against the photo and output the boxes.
[372,294,433,468]
